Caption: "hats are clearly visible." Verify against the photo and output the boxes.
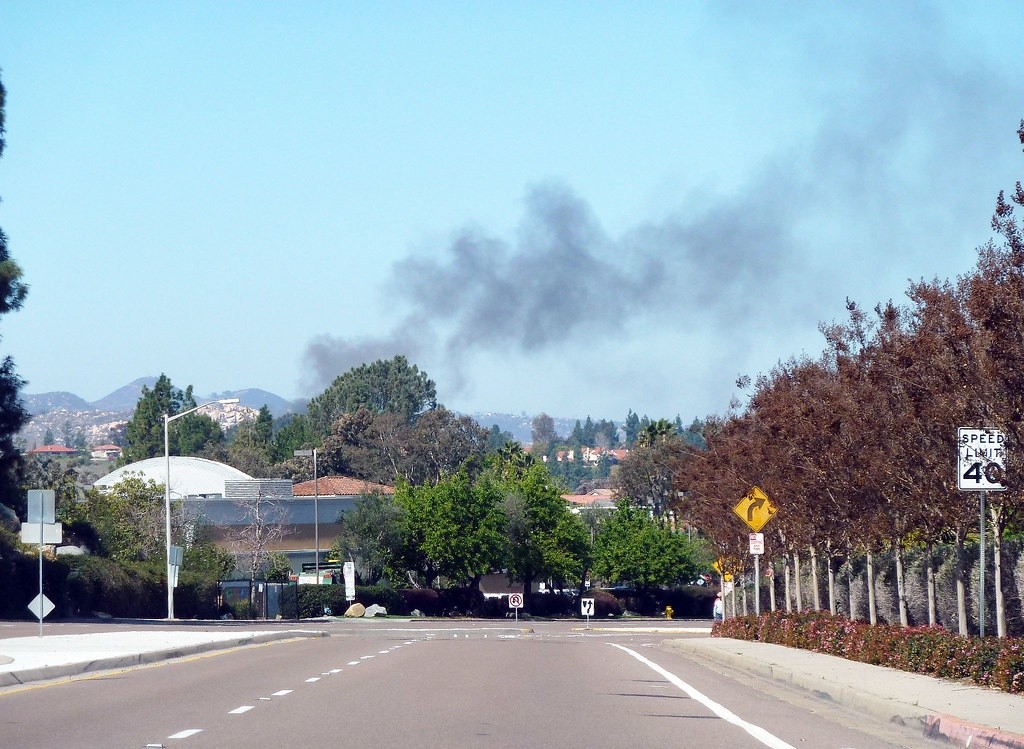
[717,592,723,598]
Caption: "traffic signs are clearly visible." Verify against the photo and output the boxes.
[957,426,1008,492]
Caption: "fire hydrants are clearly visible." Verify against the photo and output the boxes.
[665,605,672,618]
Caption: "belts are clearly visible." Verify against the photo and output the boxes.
[716,612,722,616]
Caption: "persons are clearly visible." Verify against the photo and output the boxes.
[713,592,723,621]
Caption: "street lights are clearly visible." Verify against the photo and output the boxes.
[165,398,240,618]
